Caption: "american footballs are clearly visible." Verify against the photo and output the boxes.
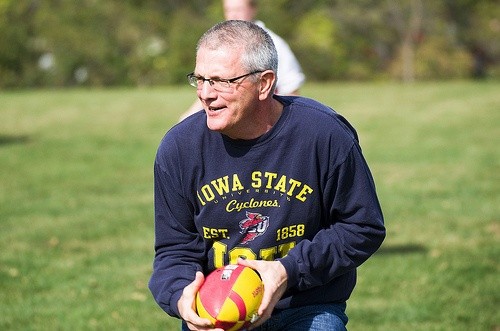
[195,265,264,328]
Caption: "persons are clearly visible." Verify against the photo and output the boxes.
[179,0,306,124]
[149,19,388,331]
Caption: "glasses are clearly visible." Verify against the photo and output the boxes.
[187,69,263,91]
